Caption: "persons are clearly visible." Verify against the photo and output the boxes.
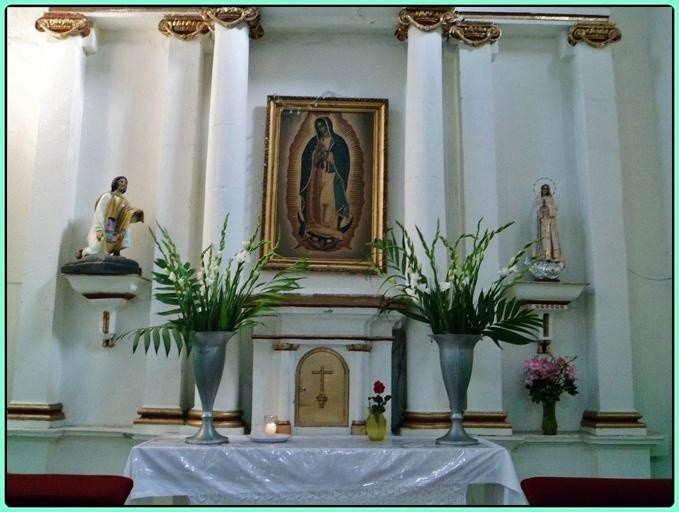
[298,114,354,248]
[528,183,564,264]
[75,176,145,261]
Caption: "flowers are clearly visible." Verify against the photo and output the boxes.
[364,213,553,353]
[522,354,578,404]
[107,211,311,357]
[367,380,392,415]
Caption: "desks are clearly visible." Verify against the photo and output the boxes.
[122,436,531,507]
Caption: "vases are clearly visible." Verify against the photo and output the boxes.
[425,333,484,446]
[184,330,235,445]
[364,412,388,441]
[541,396,558,435]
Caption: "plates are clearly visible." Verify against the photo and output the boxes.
[248,433,290,443]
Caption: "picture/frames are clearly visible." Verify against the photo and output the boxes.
[259,95,388,274]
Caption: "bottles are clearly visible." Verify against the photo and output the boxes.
[366,407,387,441]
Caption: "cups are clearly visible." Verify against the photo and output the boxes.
[264,414,278,436]
